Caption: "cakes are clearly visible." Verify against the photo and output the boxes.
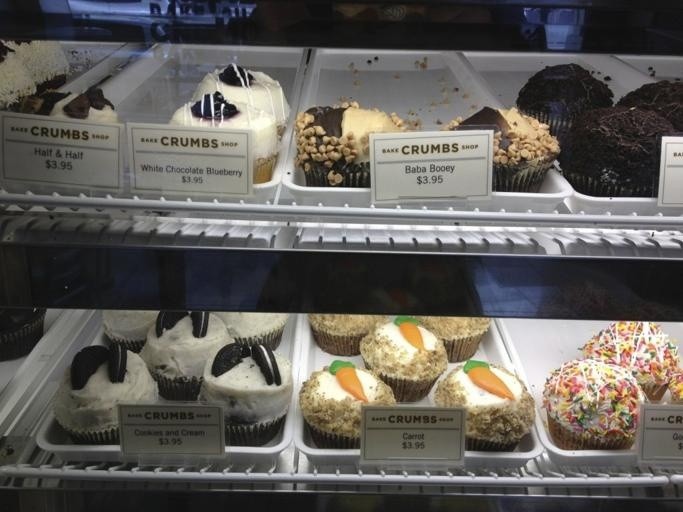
[294,101,405,187]
[441,106,562,192]
[53,310,294,448]
[0,37,119,123]
[191,62,291,140]
[516,63,683,197]
[543,321,683,448]
[0,308,47,361]
[299,312,536,451]
[167,91,282,185]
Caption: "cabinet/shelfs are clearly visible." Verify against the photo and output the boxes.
[0,187,683,501]
